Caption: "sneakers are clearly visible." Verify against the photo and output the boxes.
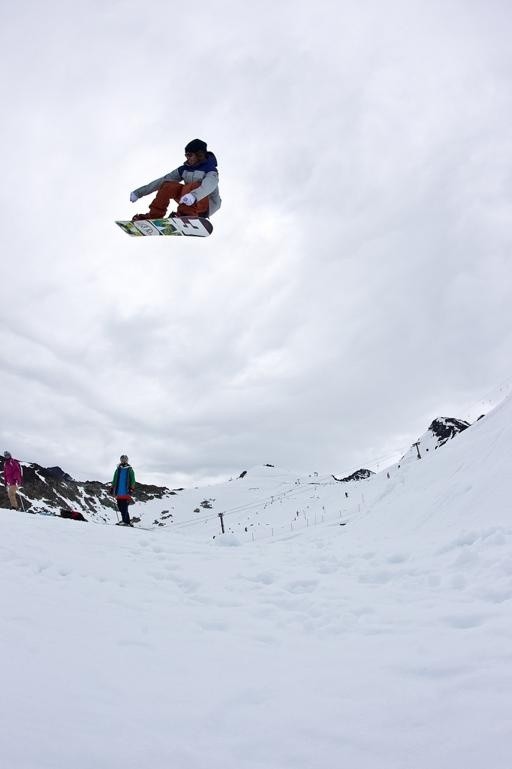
[133,213,152,221]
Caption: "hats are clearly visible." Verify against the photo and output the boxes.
[4,451,10,457]
[120,455,128,463]
[185,139,208,156]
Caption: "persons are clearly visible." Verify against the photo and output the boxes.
[128,138,223,220]
[3,452,24,509]
[112,454,138,525]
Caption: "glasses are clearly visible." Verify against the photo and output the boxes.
[184,152,195,157]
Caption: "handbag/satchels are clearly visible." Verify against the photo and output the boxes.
[61,510,85,521]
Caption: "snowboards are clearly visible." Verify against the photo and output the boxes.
[115,215,214,238]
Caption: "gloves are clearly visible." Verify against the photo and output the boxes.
[129,193,137,202]
[179,192,196,207]
